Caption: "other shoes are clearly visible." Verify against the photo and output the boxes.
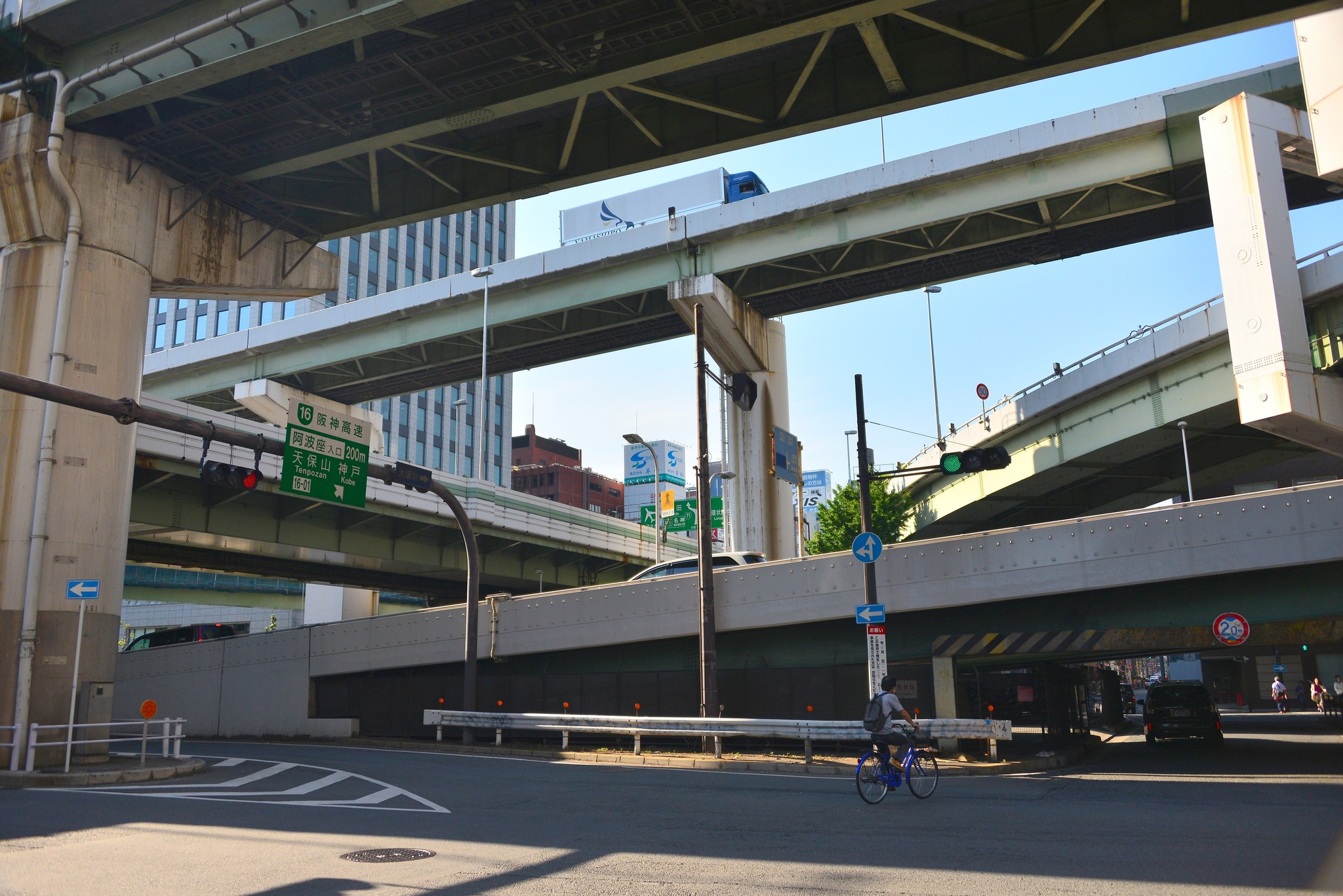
[1279,711,1283,714]
[1284,708,1286,713]
[1318,706,1321,710]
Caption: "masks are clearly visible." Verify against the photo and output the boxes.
[893,687,896,695]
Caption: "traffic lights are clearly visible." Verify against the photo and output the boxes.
[1302,644,1309,652]
[940,446,1009,475]
[205,463,263,492]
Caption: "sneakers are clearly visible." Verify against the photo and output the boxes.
[881,785,896,792]
[888,757,906,773]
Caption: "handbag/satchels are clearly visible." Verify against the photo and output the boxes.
[1273,691,1285,701]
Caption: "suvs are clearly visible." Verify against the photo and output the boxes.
[1119,681,1136,714]
[1144,674,1161,690]
[1136,679,1223,747]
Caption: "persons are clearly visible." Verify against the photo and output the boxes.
[1271,676,1288,714]
[1334,675,1343,717]
[1136,677,1146,690]
[1310,677,1327,713]
[868,675,920,792]
[1296,680,1309,712]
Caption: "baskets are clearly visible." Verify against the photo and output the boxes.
[906,729,931,748]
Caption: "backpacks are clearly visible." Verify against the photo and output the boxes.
[863,692,893,731]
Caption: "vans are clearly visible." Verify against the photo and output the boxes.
[123,622,235,654]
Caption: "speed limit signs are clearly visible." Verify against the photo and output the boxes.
[976,383,989,400]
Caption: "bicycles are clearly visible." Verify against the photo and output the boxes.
[847,722,939,804]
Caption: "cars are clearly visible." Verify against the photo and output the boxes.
[627,551,767,583]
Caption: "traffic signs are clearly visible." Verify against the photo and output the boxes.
[640,497,724,531]
[281,397,372,507]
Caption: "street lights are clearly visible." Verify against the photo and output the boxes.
[623,434,661,565]
[471,267,493,480]
[1177,421,1194,502]
[533,569,543,593]
[710,471,737,489]
[922,285,942,442]
[844,430,857,486]
[453,400,468,474]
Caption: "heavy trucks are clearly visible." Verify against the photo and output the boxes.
[559,167,769,248]
